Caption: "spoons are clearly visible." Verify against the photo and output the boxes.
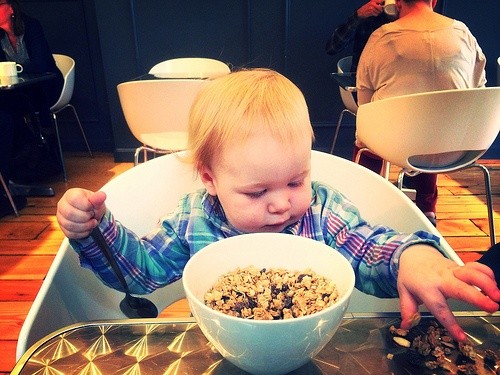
[89,225,158,319]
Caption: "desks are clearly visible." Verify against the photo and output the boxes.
[329,71,418,202]
[9,310,500,375]
[0,70,57,198]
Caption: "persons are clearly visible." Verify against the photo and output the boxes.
[326,0,487,226]
[56,69,500,341]
[0,1,63,186]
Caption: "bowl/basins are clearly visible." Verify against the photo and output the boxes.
[182,233,356,375]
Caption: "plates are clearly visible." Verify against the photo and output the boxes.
[379,322,441,375]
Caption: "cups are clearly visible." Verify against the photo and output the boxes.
[0,62,24,77]
[1,78,24,87]
[380,0,398,15]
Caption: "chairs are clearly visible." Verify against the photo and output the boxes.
[16,148,483,363]
[0,54,500,249]
